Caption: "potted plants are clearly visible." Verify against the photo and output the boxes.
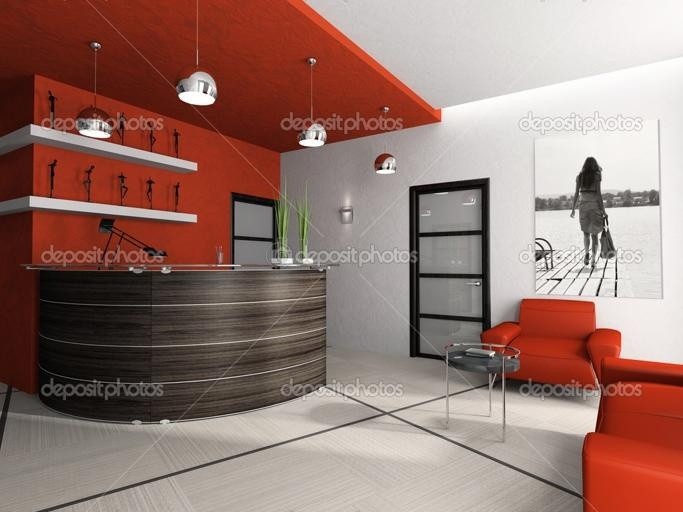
[293,182,319,265]
[265,175,293,268]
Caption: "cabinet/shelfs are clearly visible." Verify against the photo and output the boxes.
[0,122,198,223]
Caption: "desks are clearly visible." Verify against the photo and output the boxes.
[444,341,521,443]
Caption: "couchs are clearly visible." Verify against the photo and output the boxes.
[583,357,683,512]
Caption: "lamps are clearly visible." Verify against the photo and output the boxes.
[100,216,168,260]
[374,106,397,175]
[74,42,114,139]
[298,58,327,147]
[174,0,218,106]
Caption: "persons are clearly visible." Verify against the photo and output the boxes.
[569,157,608,267]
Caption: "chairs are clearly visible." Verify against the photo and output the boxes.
[479,296,621,389]
[535,237,555,270]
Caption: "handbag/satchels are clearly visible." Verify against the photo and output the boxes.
[600,215,617,258]
